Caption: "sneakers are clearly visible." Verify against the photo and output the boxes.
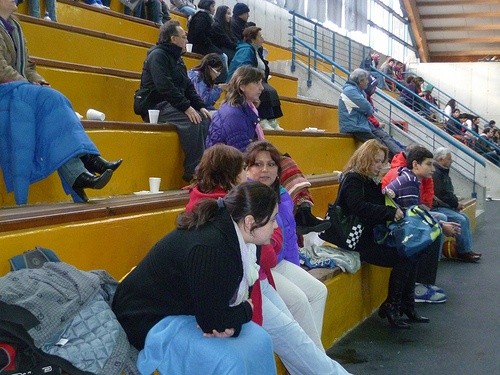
[428,284,445,293]
[414,283,447,303]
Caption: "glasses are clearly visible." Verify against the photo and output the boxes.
[208,65,221,76]
[170,34,187,39]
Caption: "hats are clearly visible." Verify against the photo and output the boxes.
[233,2,250,16]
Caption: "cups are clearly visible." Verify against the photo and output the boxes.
[148,109,160,124]
[148,177,162,193]
[86,109,105,121]
[186,43,193,52]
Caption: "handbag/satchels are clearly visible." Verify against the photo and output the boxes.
[133,89,154,114]
[9,247,60,271]
[373,205,442,258]
[318,174,363,250]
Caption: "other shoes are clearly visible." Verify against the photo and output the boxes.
[259,119,274,131]
[470,251,481,258]
[91,3,110,10]
[269,118,284,130]
[458,252,480,263]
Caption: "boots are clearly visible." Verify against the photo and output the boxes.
[380,271,410,329]
[400,280,428,323]
[294,206,332,235]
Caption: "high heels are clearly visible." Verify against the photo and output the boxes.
[72,169,113,203]
[84,155,123,175]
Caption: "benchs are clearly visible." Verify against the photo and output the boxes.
[0,0,479,375]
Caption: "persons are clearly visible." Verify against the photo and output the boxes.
[242,141,328,356]
[121,0,146,18]
[382,146,461,303]
[359,53,500,166]
[335,140,431,328]
[227,26,285,131]
[144,0,172,24]
[186,144,354,375]
[433,148,481,262]
[133,18,215,189]
[338,67,408,160]
[187,0,269,85]
[28,0,57,22]
[382,142,447,260]
[187,52,225,112]
[205,65,330,234]
[170,0,197,17]
[108,181,277,375]
[84,0,110,10]
[0,0,123,206]
